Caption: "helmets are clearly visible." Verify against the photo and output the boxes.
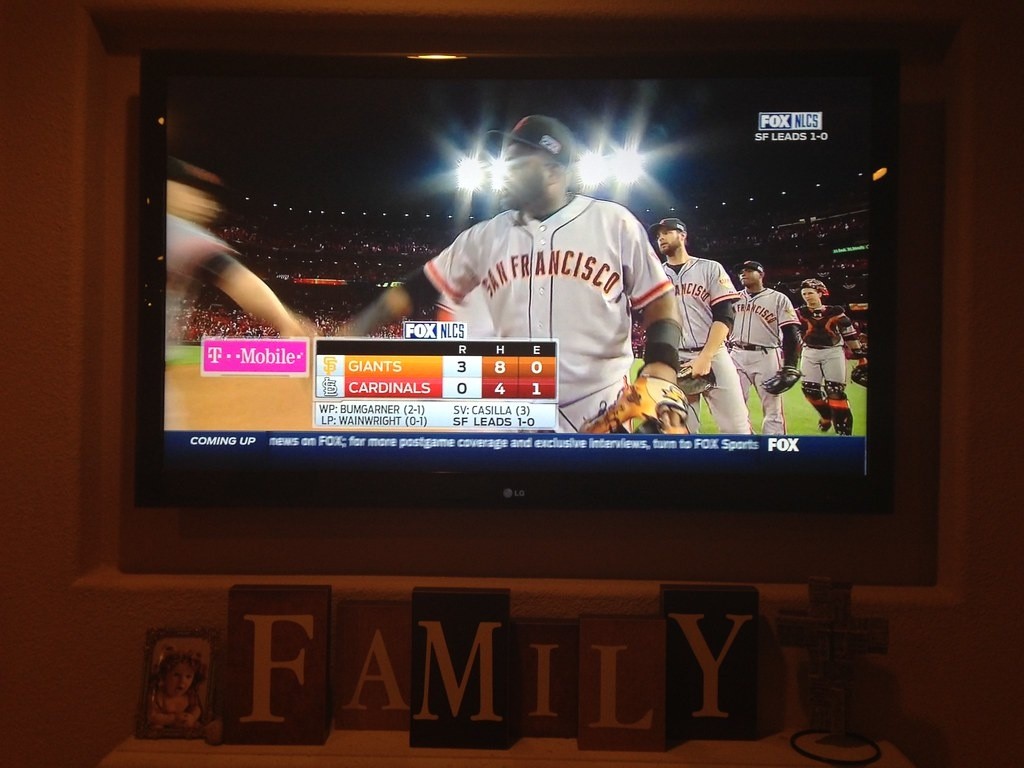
[801,278,829,296]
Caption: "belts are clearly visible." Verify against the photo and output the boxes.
[734,343,764,351]
[679,347,703,352]
[807,344,832,349]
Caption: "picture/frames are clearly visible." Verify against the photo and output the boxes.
[136,623,222,738]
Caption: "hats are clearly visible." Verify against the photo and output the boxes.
[646,218,686,232]
[483,115,572,165]
[733,261,764,273]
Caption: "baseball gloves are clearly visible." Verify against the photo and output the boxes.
[762,365,802,395]
[576,373,693,434]
[850,363,870,389]
[675,359,717,398]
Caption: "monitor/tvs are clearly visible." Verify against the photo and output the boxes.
[135,51,896,517]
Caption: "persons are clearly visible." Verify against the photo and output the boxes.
[646,219,753,435]
[165,107,865,434]
